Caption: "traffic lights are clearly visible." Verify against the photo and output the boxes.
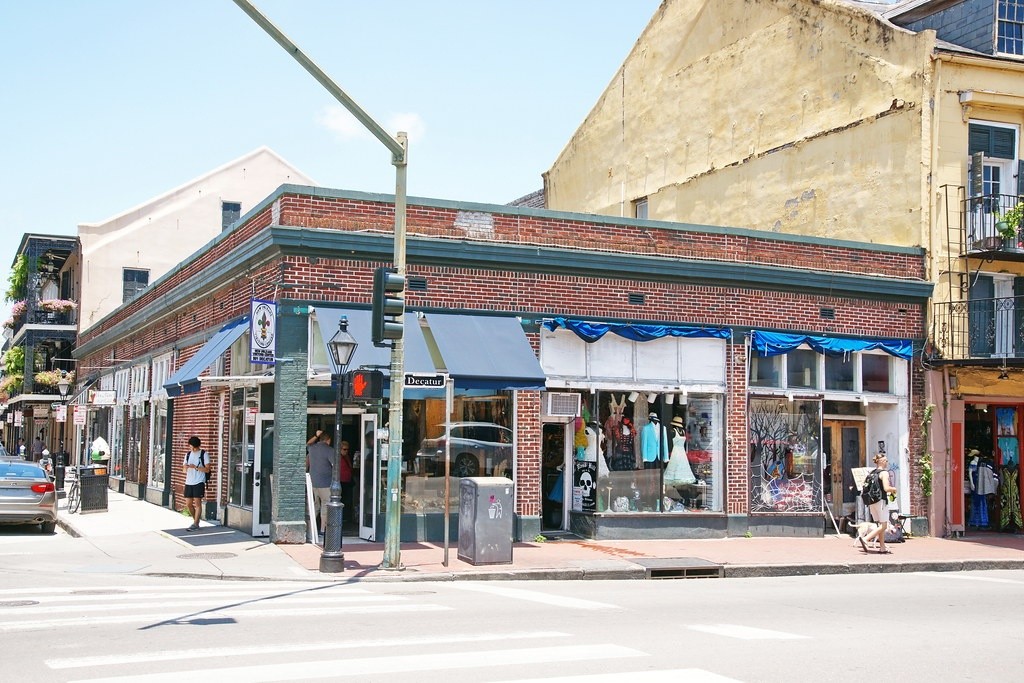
[351,369,385,401]
[370,266,406,344]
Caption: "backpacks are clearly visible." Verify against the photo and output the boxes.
[862,469,886,506]
[186,449,211,480]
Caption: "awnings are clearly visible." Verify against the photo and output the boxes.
[425,313,546,391]
[313,307,436,391]
[163,314,274,397]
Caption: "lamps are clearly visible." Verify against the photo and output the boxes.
[664,393,675,405]
[54,338,62,350]
[735,354,747,364]
[646,391,658,404]
[627,391,640,403]
[996,367,1011,379]
[40,272,48,283]
[46,260,54,272]
[679,393,689,406]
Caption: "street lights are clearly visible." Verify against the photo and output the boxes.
[55,369,71,491]
[317,313,359,573]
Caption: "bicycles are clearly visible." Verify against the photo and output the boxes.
[64,468,82,515]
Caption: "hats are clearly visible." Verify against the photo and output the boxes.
[670,416,683,428]
[968,450,980,456]
[648,412,660,421]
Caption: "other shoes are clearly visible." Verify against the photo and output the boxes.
[319,531,324,536]
[186,524,199,531]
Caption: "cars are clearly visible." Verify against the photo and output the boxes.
[0,441,59,534]
[232,441,255,476]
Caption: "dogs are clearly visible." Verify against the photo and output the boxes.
[848,520,897,549]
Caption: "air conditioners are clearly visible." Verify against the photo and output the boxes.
[541,392,581,418]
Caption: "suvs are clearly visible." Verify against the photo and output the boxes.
[415,421,513,480]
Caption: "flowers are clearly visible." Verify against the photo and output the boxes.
[37,298,78,313]
[32,369,76,388]
[992,201,1024,237]
[0,299,29,330]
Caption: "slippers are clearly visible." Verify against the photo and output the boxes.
[859,537,868,552]
[878,549,891,553]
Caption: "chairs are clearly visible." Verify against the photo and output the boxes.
[899,514,918,539]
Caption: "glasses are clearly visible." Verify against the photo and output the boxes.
[341,448,349,451]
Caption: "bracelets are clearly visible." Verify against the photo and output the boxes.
[195,466,198,470]
[315,435,318,438]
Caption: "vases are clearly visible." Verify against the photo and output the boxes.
[43,304,50,309]
[1004,237,1017,248]
[985,236,1001,249]
[995,220,1009,232]
[68,306,71,311]
[23,307,27,313]
[15,315,20,321]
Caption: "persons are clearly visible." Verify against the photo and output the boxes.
[604,392,627,458]
[364,431,374,527]
[16,438,27,456]
[32,437,47,462]
[641,413,670,469]
[968,450,988,526]
[584,423,610,477]
[183,437,211,531]
[306,430,354,537]
[663,416,696,485]
[859,454,897,554]
[611,417,637,469]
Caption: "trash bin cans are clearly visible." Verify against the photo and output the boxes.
[458,476,515,566]
[79,467,108,513]
